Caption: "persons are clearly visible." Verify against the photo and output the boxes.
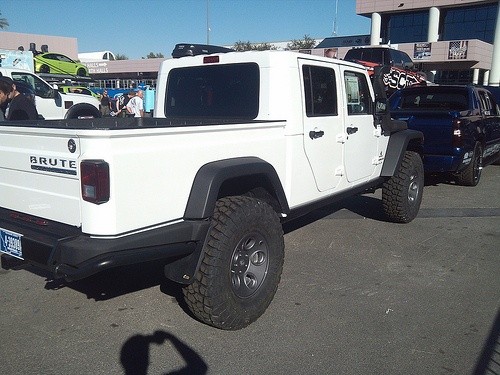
[101,89,110,117]
[122,90,144,118]
[0,76,38,121]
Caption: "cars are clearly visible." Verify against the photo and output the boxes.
[0,68,102,120]
[33,52,89,77]
[58,86,101,99]
[343,47,424,94]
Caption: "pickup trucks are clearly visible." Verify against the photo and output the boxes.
[0,49,428,330]
[382,82,499,186]
